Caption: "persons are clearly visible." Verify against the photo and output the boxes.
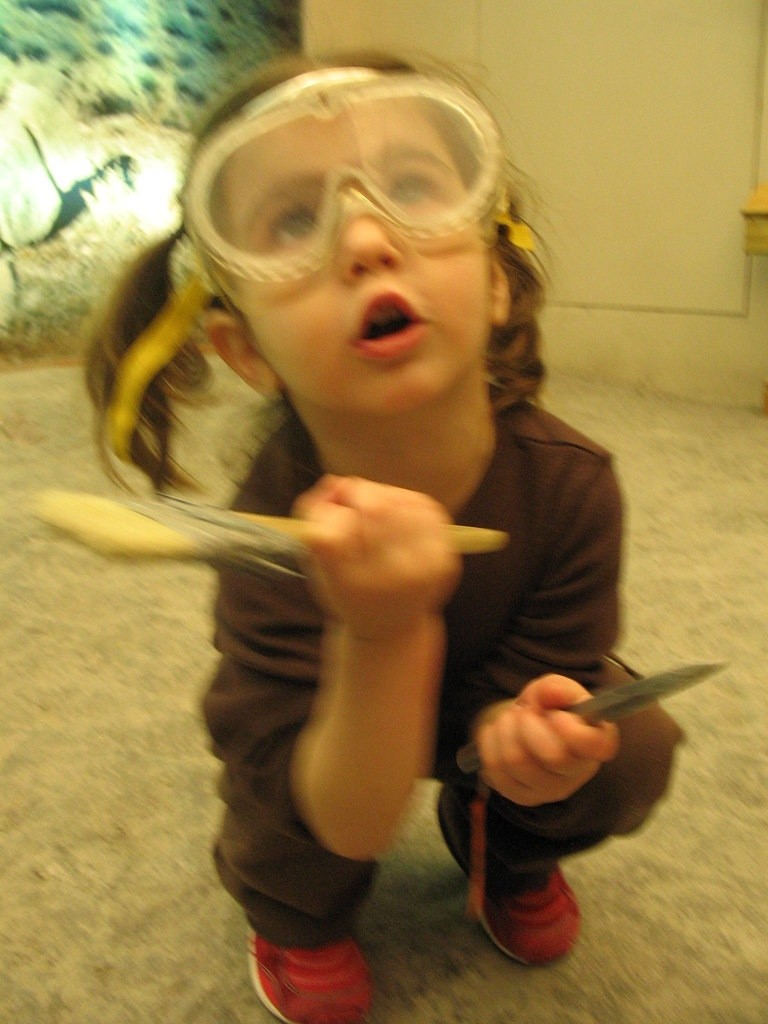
[81,50,690,1024]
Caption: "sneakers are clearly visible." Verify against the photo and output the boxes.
[437,786,583,964]
[246,924,375,1024]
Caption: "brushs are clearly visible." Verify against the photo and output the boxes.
[23,481,512,567]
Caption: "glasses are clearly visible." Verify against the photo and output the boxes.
[168,67,509,292]
